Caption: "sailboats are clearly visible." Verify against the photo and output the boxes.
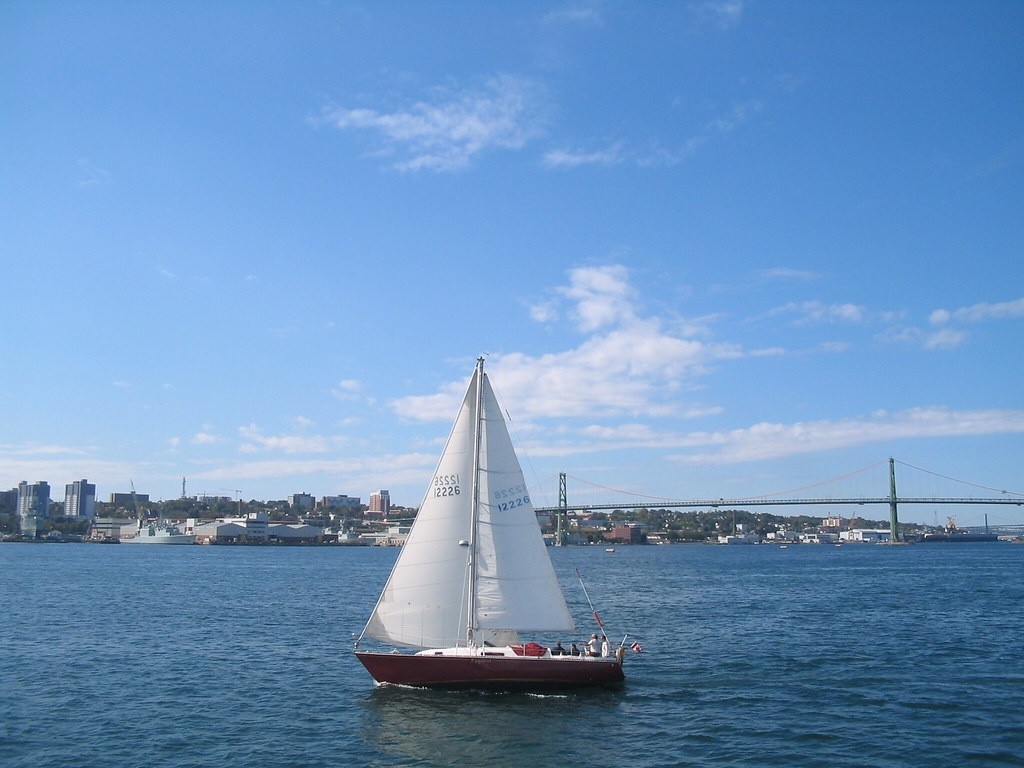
[348,352,641,690]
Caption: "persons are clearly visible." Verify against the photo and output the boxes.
[583,634,601,656]
[567,643,581,656]
[600,635,610,657]
[551,641,567,656]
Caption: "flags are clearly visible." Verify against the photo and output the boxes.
[631,641,642,653]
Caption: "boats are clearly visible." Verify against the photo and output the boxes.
[119,517,198,544]
